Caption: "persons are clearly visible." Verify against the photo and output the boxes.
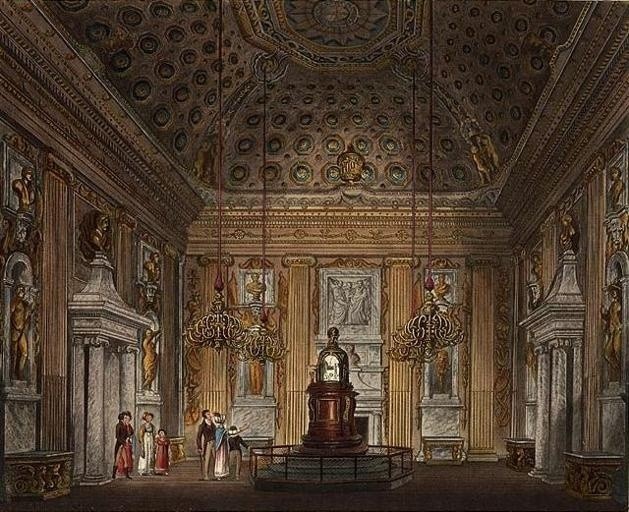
[26,288,44,376]
[138,411,155,476]
[209,144,226,185]
[247,273,267,301]
[143,250,164,278]
[227,425,249,482]
[558,213,576,252]
[197,410,215,478]
[193,140,212,179]
[89,214,113,252]
[141,327,162,390]
[470,143,492,186]
[213,411,230,480]
[607,166,625,205]
[318,274,453,402]
[596,284,622,380]
[112,410,134,481]
[154,430,171,475]
[13,166,43,214]
[481,133,501,171]
[9,282,28,382]
[116,409,134,476]
[529,253,543,280]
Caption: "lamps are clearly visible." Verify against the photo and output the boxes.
[179,0,290,361]
[386,0,464,365]
